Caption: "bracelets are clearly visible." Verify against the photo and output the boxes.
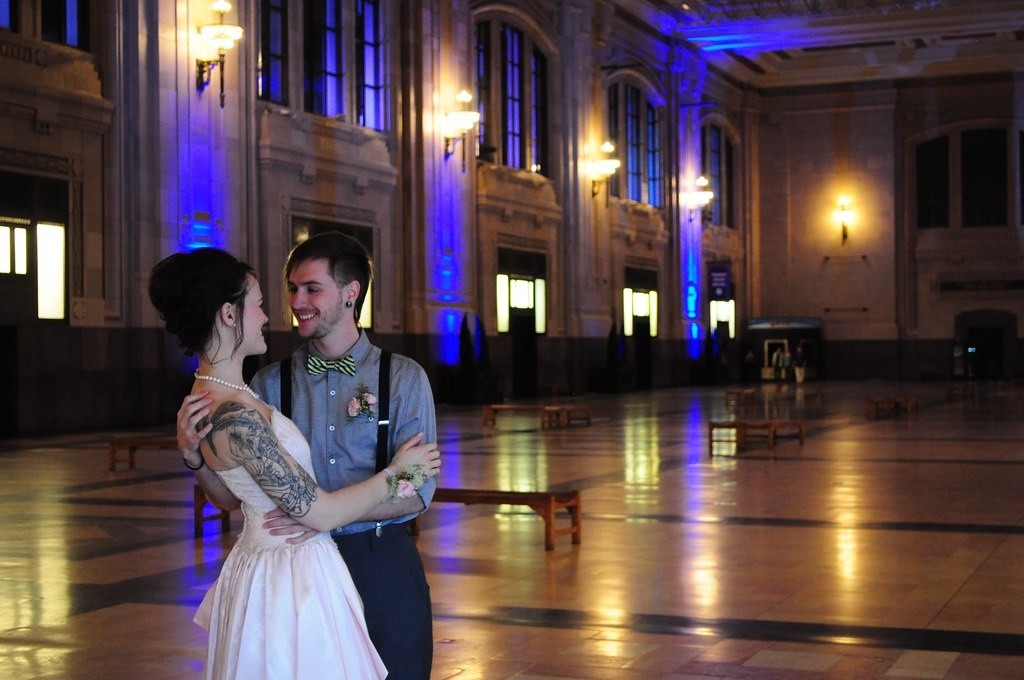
[384,463,426,502]
[183,453,205,470]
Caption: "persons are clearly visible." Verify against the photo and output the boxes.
[176,232,438,680]
[147,248,441,680]
[772,347,805,384]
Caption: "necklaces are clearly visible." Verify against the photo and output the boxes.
[195,368,260,399]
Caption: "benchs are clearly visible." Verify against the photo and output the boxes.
[482,405,592,426]
[414,485,582,548]
[195,485,234,537]
[707,383,975,453]
[109,437,180,467]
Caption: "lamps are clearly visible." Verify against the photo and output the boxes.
[194,0,244,108]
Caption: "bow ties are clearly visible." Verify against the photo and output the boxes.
[307,355,356,377]
